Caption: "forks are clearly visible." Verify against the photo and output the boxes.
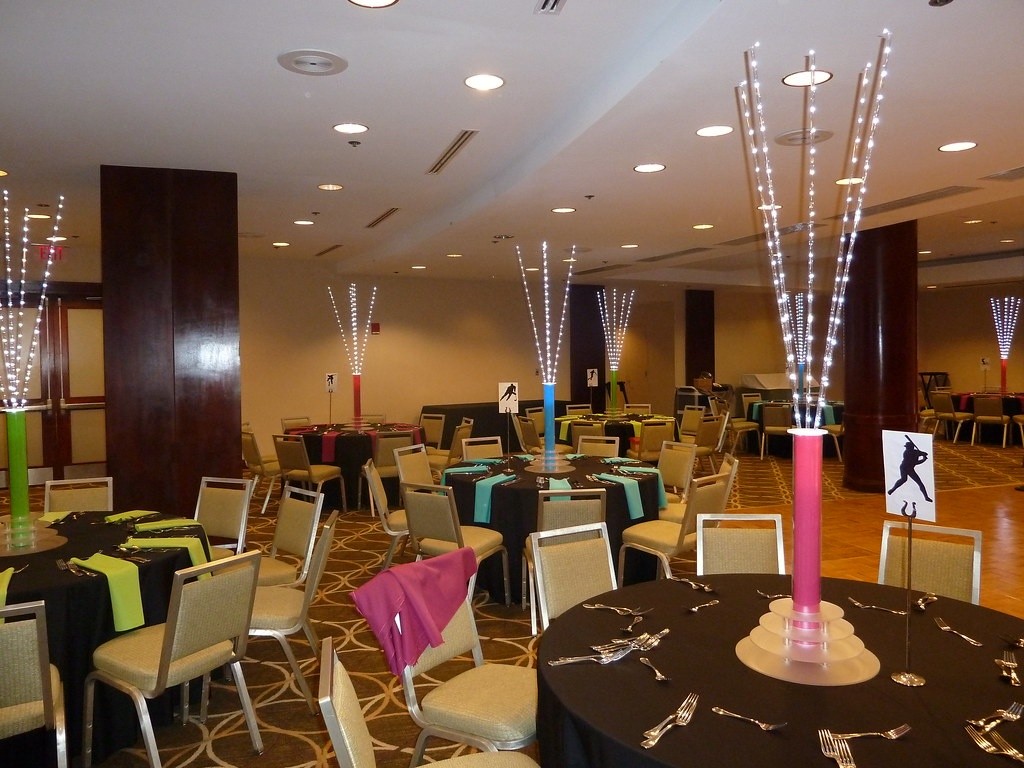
[1004,651,1022,686]
[999,633,1024,647]
[671,576,719,592]
[546,603,670,666]
[641,700,697,748]
[848,596,907,615]
[712,707,787,731]
[643,693,701,738]
[831,723,911,740]
[757,589,792,598]
[934,617,983,646]
[599,457,656,476]
[451,455,524,485]
[535,475,616,487]
[911,592,936,610]
[966,709,1005,727]
[979,702,1024,735]
[682,600,719,612]
[819,730,843,768]
[965,725,1024,763]
[640,658,672,681]
[832,740,856,768]
[51,510,198,577]
[989,731,1024,762]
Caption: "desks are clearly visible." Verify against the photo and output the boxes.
[918,372,948,410]
[419,399,574,455]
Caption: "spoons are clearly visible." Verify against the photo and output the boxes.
[995,659,1011,680]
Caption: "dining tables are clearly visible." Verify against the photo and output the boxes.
[441,451,662,608]
[281,422,427,513]
[943,392,1024,447]
[745,399,846,460]
[553,412,680,468]
[0,511,212,768]
[536,572,1024,768]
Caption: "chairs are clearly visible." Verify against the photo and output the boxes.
[44,476,113,512]
[0,599,69,768]
[198,509,339,725]
[371,550,539,768]
[194,476,253,561]
[83,548,266,768]
[317,635,542,768]
[242,374,1024,635]
